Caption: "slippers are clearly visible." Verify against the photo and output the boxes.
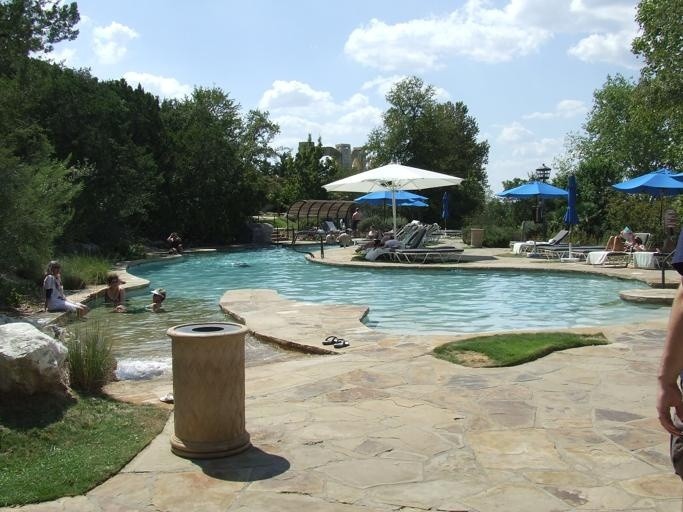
[322,336,341,344]
[334,339,350,348]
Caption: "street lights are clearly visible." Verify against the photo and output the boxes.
[535,163,552,242]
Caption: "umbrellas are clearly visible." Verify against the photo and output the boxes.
[353,197,429,222]
[440,192,450,236]
[497,178,577,252]
[353,191,429,224]
[613,164,683,240]
[563,172,579,257]
[320,158,465,238]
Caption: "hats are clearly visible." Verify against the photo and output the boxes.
[150,289,164,298]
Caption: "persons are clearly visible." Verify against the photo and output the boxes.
[38,259,90,317]
[105,274,126,303]
[658,229,683,481]
[113,305,127,312]
[367,224,401,248]
[149,288,167,310]
[326,219,351,247]
[168,232,184,254]
[623,236,646,252]
[604,228,625,251]
[351,208,362,231]
[656,227,676,254]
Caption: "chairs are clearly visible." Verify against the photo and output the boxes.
[304,218,464,264]
[508,227,677,270]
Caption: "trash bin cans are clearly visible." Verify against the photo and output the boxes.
[166,321,252,460]
[471,229,484,248]
